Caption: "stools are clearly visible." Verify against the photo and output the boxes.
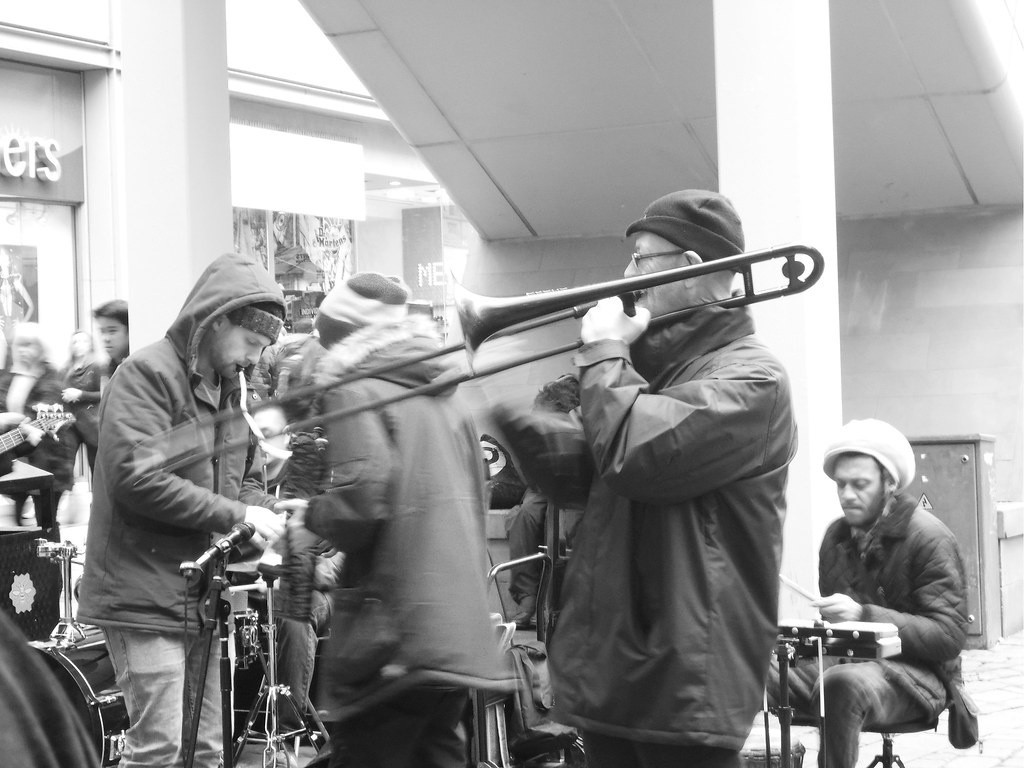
[842,713,940,768]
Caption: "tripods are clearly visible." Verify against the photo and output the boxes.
[236,563,320,768]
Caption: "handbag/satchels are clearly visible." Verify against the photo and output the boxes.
[503,639,578,757]
[945,678,981,750]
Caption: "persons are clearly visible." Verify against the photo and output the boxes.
[763,417,968,768]
[0,297,131,550]
[246,332,587,768]
[490,187,797,768]
[271,269,524,768]
[72,252,290,768]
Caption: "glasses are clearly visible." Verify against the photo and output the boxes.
[631,250,685,268]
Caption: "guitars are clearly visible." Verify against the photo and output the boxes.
[0,403,78,455]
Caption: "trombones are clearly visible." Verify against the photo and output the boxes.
[134,242,826,481]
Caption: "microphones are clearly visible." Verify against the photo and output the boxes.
[184,522,256,578]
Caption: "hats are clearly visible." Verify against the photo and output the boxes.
[226,302,285,346]
[624,189,744,270]
[821,418,916,495]
[315,271,408,342]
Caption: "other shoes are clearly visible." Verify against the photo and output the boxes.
[271,732,299,768]
